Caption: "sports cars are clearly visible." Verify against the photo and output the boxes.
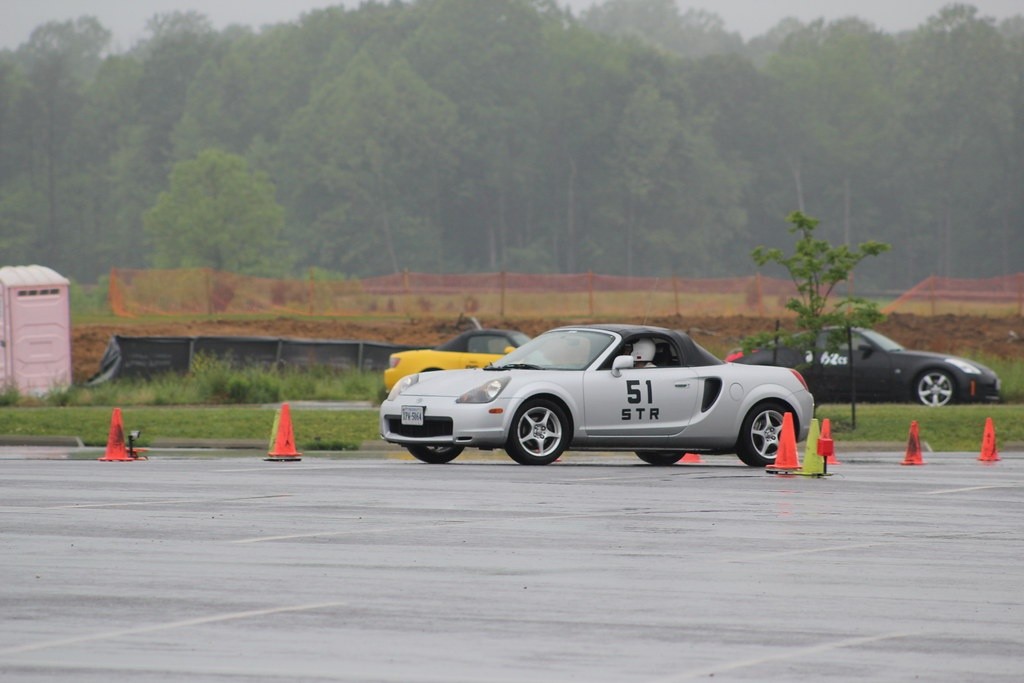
[377,321,815,466]
[381,328,558,395]
[723,325,1003,406]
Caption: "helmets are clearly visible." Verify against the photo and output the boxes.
[625,337,656,361]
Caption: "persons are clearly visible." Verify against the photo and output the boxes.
[624,336,658,369]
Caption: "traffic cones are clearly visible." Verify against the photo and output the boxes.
[96,406,135,462]
[793,416,827,475]
[820,417,841,465]
[267,402,303,458]
[975,417,1002,462]
[678,454,704,464]
[764,411,803,471]
[262,408,281,461]
[900,420,926,465]
